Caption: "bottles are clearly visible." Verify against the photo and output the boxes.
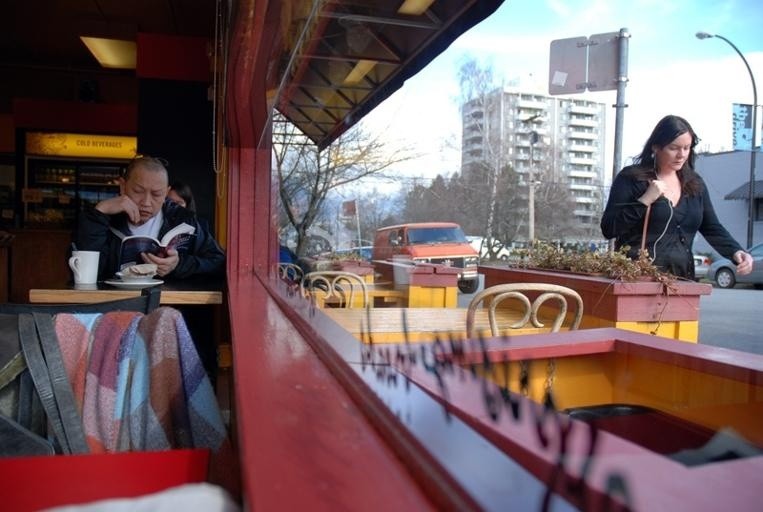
[34,169,74,182]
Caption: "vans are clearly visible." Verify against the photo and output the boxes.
[373,221,481,294]
[466,235,509,261]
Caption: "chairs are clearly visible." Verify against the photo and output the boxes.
[0,280,162,461]
[274,262,305,285]
[462,280,585,338]
[297,270,369,312]
[14,308,240,504]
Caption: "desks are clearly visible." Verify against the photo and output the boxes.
[295,284,403,311]
[314,303,574,347]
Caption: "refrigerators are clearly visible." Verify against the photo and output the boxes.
[17,127,141,226]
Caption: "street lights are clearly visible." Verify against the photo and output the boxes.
[695,30,758,250]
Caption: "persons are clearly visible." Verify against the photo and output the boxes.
[600,115,753,281]
[165,182,208,228]
[280,245,305,278]
[68,157,226,290]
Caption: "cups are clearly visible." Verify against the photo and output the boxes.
[67,249,100,286]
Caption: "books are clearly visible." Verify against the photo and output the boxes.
[118,223,196,268]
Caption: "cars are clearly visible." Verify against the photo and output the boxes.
[707,244,762,289]
[310,239,373,261]
[692,255,710,283]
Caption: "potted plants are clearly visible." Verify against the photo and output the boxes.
[472,236,716,349]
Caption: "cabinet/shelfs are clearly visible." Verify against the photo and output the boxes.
[19,151,133,233]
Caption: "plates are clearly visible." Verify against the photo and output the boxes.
[104,278,163,289]
